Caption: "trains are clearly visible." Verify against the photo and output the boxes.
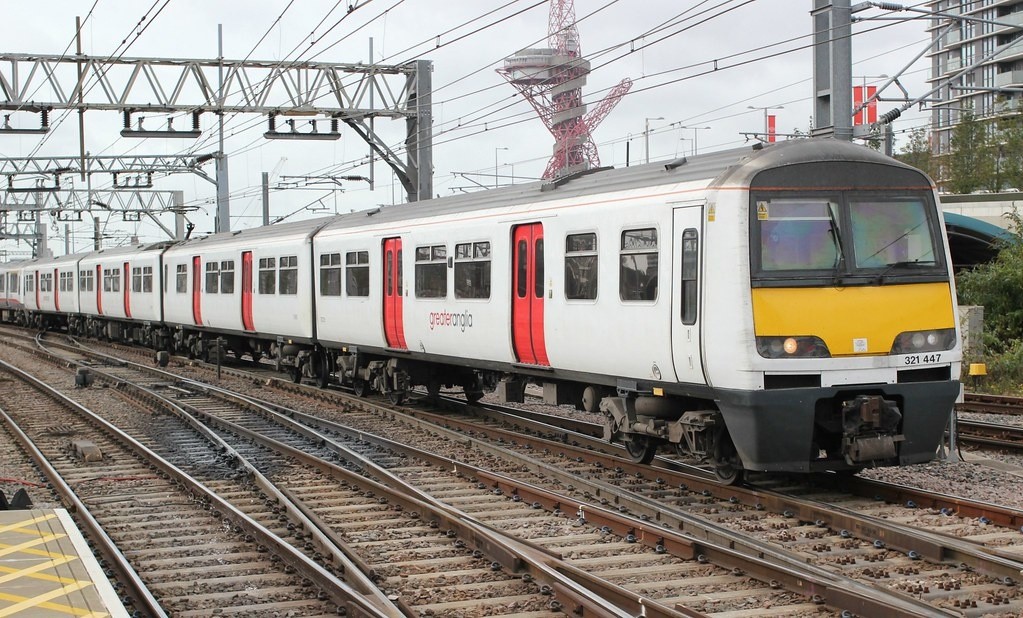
[0,136,964,487]
